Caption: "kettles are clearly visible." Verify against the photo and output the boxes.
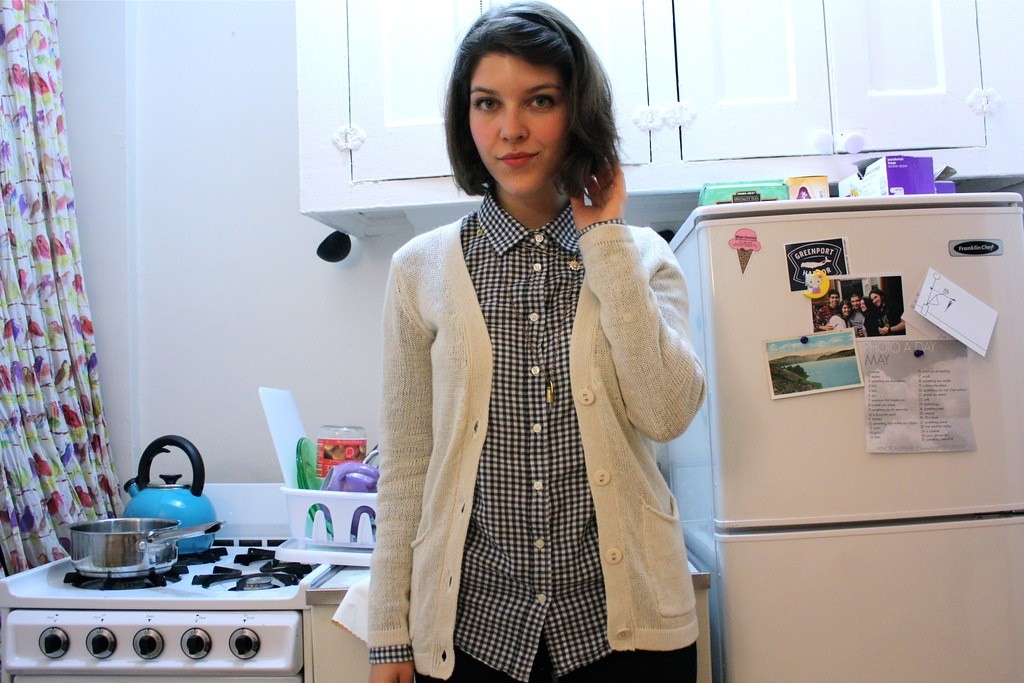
[119,435,217,554]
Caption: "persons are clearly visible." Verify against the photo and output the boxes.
[815,288,905,336]
[367,2,705,683]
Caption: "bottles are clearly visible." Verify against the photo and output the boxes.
[316,423,368,481]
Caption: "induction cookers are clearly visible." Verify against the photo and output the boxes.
[0,546,311,677]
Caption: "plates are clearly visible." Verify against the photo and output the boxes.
[297,437,333,535]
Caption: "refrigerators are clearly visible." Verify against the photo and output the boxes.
[662,193,1024,683]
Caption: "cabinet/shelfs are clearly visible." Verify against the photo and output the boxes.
[293,0,1024,239]
[305,565,713,683]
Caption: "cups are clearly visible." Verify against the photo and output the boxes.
[320,463,379,494]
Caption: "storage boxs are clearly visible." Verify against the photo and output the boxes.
[202,386,308,542]
[698,178,789,205]
[281,484,378,547]
[839,155,935,198]
[935,166,956,194]
[788,175,829,200]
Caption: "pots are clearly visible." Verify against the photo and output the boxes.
[69,517,228,578]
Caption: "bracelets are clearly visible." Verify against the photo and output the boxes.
[888,327,891,334]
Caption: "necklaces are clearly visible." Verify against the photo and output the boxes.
[519,243,580,271]
[494,255,575,404]
